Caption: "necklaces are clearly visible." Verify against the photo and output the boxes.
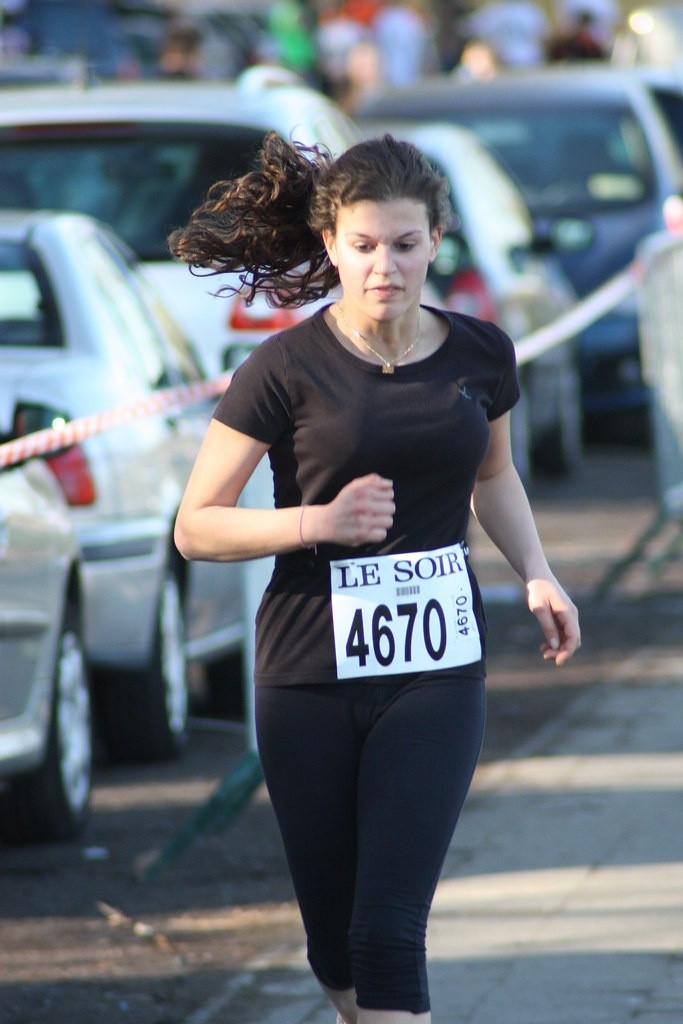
[337,303,422,374]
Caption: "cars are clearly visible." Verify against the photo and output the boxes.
[1,202,259,760]
[1,1,681,488]
[1,425,101,841]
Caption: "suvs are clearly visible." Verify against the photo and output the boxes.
[1,65,370,459]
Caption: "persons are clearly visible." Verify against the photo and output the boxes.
[170,132,580,1024]
[0,0,617,112]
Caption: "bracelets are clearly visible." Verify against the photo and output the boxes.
[296,503,317,554]
